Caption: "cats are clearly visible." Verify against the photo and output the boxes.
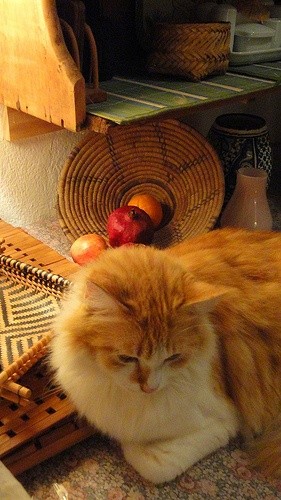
[40,226,280,496]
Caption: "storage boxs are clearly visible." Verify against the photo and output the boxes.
[144,22,232,83]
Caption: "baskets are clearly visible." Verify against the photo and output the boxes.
[145,22,231,82]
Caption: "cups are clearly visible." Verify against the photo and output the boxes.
[263,18,281,47]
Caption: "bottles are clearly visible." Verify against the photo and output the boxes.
[220,167,273,231]
[211,0,237,51]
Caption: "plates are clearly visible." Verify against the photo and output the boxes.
[229,47,281,65]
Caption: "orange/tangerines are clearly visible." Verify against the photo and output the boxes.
[127,193,163,228]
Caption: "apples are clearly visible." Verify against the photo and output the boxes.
[69,234,107,266]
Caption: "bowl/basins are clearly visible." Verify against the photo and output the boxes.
[233,23,275,52]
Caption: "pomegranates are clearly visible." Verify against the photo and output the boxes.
[107,206,154,249]
